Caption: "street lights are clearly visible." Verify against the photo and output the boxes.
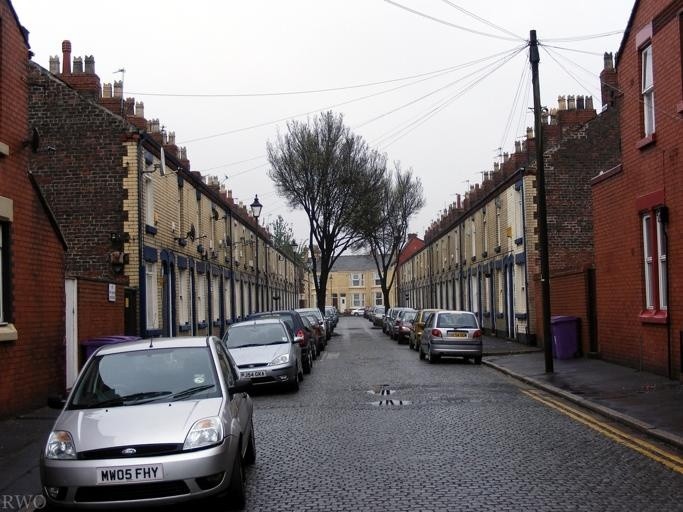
[290,238,298,309]
[328,273,332,306]
[248,193,263,312]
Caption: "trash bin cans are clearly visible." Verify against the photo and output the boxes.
[549,315,581,360]
[79,335,143,361]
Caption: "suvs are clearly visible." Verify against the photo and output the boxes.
[246,309,314,374]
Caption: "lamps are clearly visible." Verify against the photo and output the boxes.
[140,145,167,178]
[174,222,196,242]
[11,128,41,154]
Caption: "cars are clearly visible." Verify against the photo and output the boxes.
[418,311,484,365]
[219,318,304,393]
[40,334,257,510]
[293,304,338,360]
[407,309,445,351]
[363,303,417,345]
[350,307,364,316]
